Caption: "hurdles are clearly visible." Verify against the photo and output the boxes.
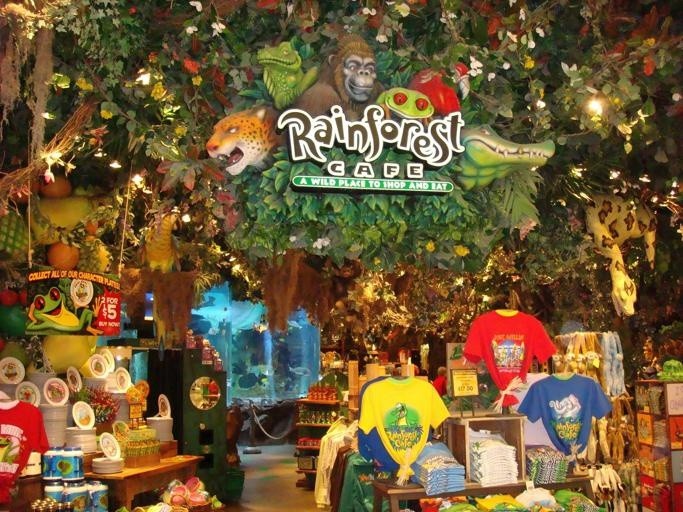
[25,194,111,248]
[41,335,91,374]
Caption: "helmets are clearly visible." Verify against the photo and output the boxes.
[0,356,26,384]
[40,403,68,447]
[66,366,82,392]
[88,354,109,379]
[43,377,69,406]
[157,393,171,419]
[147,417,174,443]
[15,380,41,407]
[114,366,131,393]
[71,400,96,430]
[100,349,115,373]
[92,458,124,475]
[99,431,122,461]
[65,427,98,455]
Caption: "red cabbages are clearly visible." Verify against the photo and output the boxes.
[77,239,113,275]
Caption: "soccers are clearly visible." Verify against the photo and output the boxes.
[0,276,42,375]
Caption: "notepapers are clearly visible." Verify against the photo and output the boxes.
[0,209,32,266]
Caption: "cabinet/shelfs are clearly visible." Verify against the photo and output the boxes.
[0,347,244,512]
[293,330,683,512]
[41,447,110,512]
